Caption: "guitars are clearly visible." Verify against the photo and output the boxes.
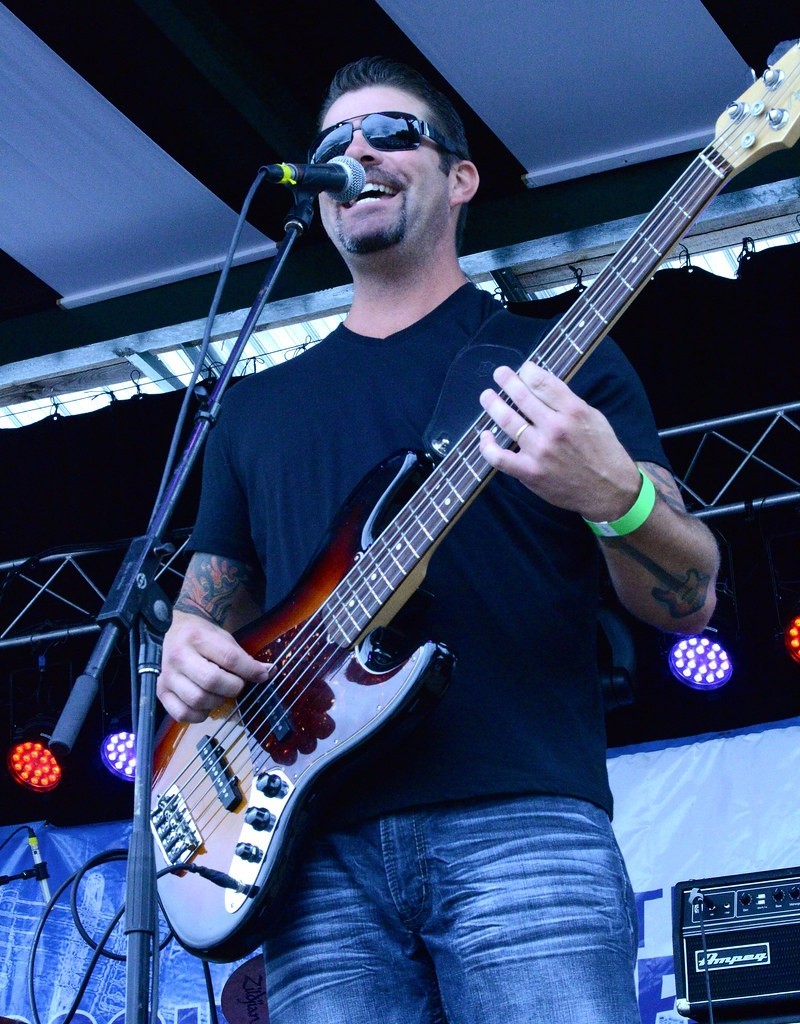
[147,38,800,966]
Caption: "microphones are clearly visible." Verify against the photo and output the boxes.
[258,155,366,203]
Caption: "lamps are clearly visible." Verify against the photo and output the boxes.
[98,670,139,782]
[6,676,76,793]
[660,546,738,688]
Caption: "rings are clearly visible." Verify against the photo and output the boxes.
[515,424,529,441]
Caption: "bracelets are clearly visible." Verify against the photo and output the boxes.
[581,470,656,537]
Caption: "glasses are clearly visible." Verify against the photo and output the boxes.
[307,111,462,168]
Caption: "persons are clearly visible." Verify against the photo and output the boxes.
[156,57,721,1024]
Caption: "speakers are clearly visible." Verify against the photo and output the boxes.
[673,866,800,1024]
